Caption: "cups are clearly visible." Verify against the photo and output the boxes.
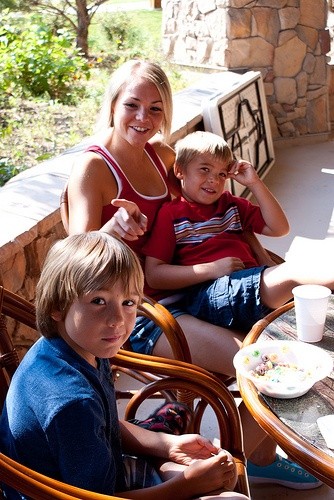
[292,284,332,342]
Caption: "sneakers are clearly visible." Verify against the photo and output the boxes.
[247,453,323,489]
[129,402,193,434]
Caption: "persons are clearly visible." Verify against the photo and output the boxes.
[141,130,333,334]
[63,59,326,490]
[1,230,252,500]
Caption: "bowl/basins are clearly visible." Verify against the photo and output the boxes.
[232,340,333,399]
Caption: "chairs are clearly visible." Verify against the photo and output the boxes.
[0,285,249,500]
[57,178,243,444]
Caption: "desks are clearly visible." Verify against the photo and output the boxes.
[235,294,334,491]
